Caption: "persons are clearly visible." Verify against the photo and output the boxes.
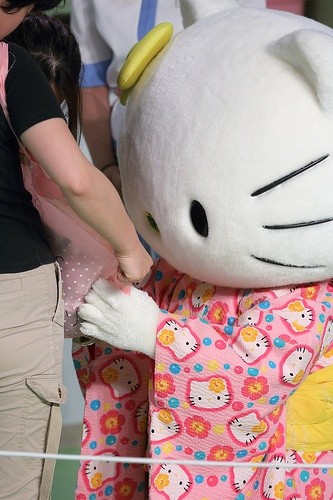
[0,0,266,500]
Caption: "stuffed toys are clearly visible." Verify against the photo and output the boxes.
[70,8,333,500]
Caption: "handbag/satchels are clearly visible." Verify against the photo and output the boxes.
[0,42,130,338]
